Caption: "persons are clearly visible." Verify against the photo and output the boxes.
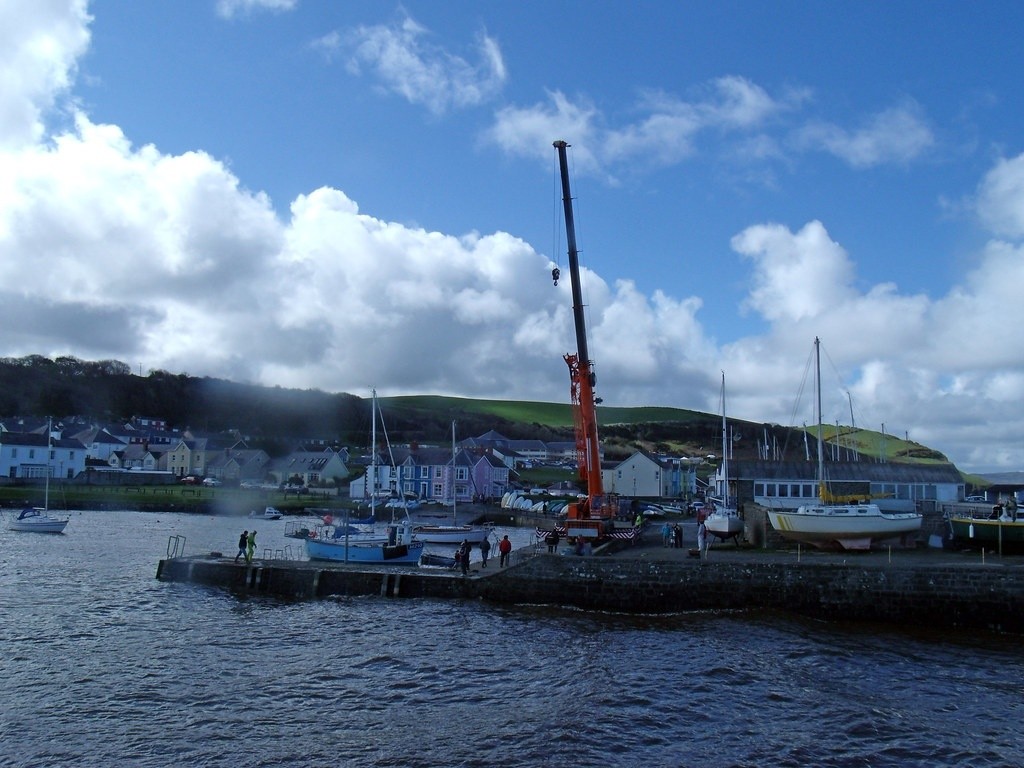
[1006,496,1017,523]
[662,522,683,548]
[697,520,707,551]
[246,530,257,561]
[459,539,472,576]
[235,531,250,563]
[546,529,559,553]
[542,503,547,519]
[499,535,511,568]
[480,536,490,568]
[452,550,460,570]
[575,534,584,555]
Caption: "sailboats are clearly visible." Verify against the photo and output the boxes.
[7,416,74,534]
[319,391,422,548]
[701,371,746,538]
[767,336,925,547]
[410,419,496,543]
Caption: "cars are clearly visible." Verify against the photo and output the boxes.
[514,489,589,497]
[404,490,418,499]
[240,480,305,491]
[644,500,705,516]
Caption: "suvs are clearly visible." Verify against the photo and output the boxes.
[181,477,200,486]
[966,496,997,502]
[371,488,399,499]
[203,477,222,487]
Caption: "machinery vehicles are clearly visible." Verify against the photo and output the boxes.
[546,139,651,547]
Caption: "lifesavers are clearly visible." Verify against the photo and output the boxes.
[324,516,332,524]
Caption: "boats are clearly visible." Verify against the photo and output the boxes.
[304,538,427,563]
[947,513,1024,549]
[248,507,284,521]
[352,498,454,509]
[421,553,469,568]
[500,491,568,515]
